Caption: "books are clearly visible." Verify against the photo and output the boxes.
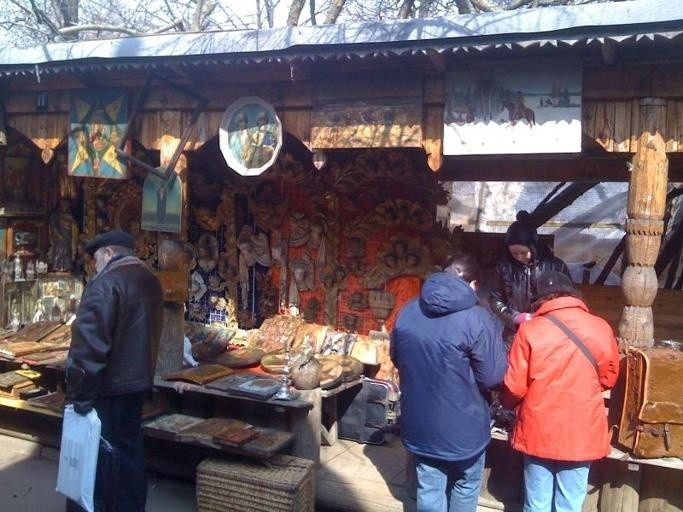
[0,316,74,412]
[139,363,283,447]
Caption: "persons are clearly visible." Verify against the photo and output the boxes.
[489,213,571,388]
[63,231,164,511]
[227,110,252,163]
[499,269,621,510]
[241,111,278,171]
[386,255,505,510]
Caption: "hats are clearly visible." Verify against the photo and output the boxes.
[503,209,537,249]
[82,229,133,256]
[528,270,580,304]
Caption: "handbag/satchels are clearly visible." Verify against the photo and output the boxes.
[61,415,123,511]
[53,401,100,512]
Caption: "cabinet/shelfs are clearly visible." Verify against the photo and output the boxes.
[0,341,366,471]
